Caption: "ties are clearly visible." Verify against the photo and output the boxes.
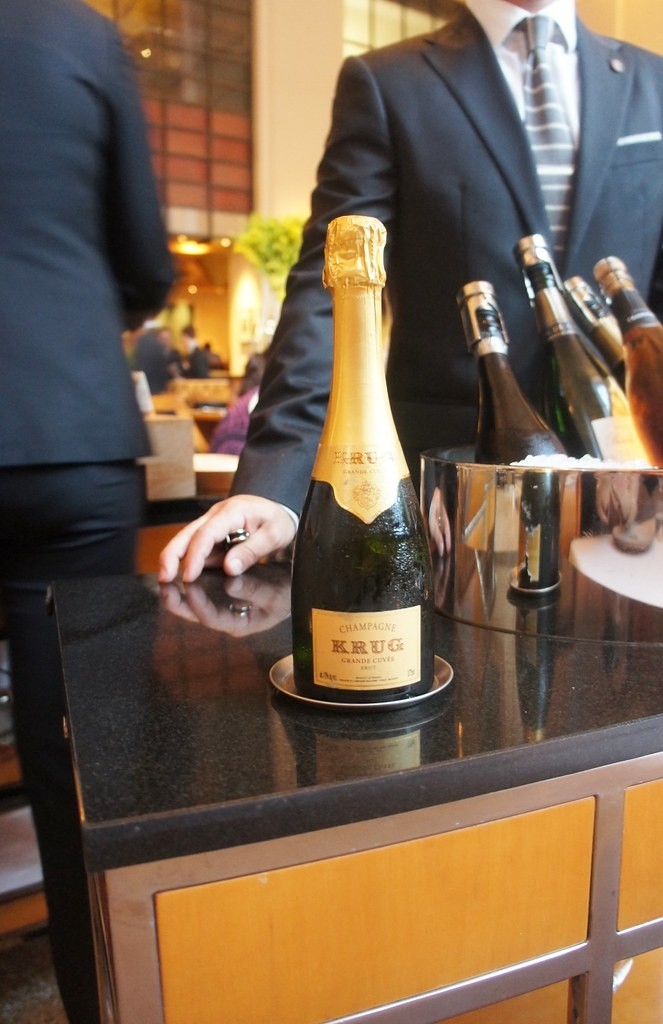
[520,15,577,273]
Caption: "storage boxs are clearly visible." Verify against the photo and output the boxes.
[48,469,662,1024]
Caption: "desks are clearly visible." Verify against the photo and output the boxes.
[192,455,239,500]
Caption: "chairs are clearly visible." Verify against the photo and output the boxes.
[141,416,197,501]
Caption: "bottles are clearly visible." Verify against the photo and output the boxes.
[594,250,663,474]
[455,276,569,470]
[510,227,649,468]
[286,209,435,707]
[563,270,629,389]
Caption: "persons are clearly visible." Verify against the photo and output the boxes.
[163,0,663,597]
[207,353,267,459]
[131,321,181,390]
[167,328,222,379]
[0,2,183,1024]
